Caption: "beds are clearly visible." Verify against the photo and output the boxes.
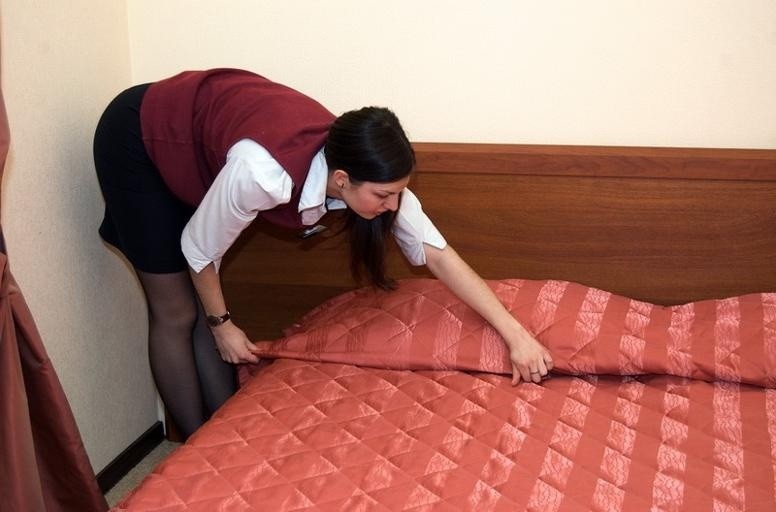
[108,142,776,512]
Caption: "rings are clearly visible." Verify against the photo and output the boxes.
[530,371,539,374]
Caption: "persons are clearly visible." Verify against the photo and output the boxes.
[91,66,554,444]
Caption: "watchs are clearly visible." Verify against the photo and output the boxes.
[206,311,230,327]
